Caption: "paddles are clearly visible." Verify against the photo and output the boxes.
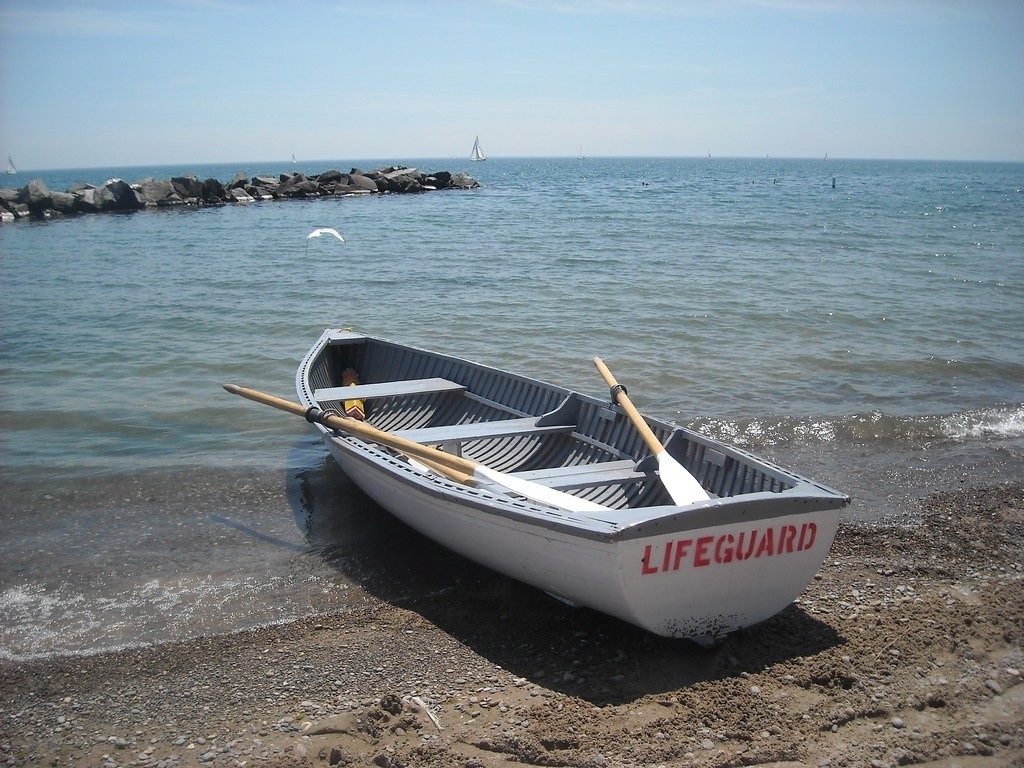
[223,381,612,518]
[594,353,713,504]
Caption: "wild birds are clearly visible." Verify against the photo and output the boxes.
[304,228,346,259]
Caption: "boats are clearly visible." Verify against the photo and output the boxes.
[296,328,852,641]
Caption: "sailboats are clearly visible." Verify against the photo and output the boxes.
[469,136,488,161]
[290,153,297,164]
[6,156,19,174]
[576,145,585,159]
